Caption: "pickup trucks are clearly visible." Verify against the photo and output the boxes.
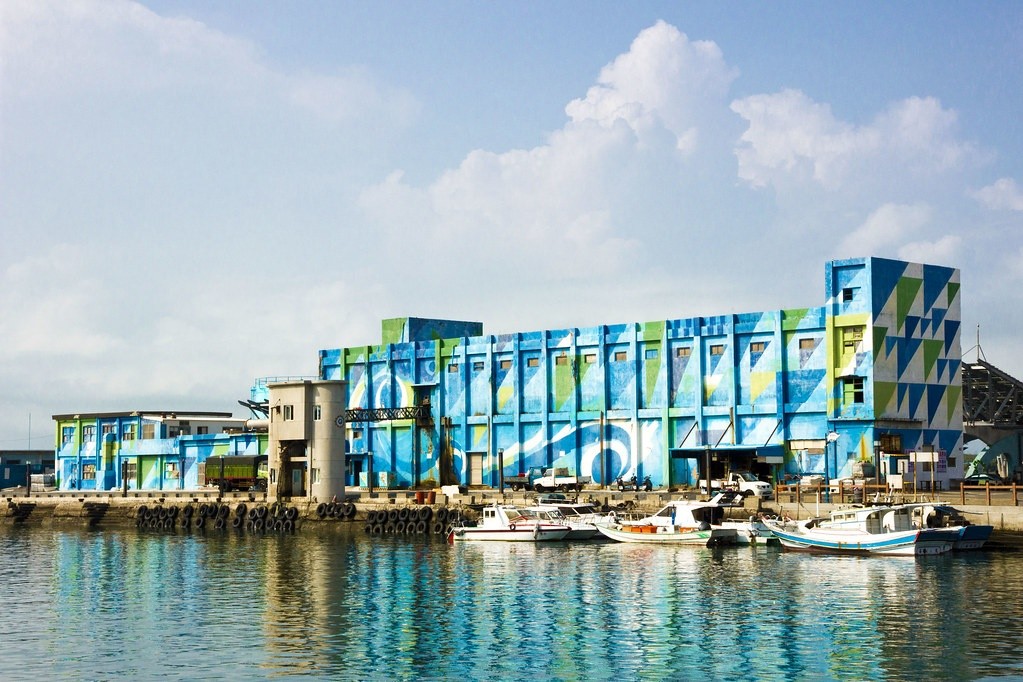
[699,471,773,501]
[533,467,592,493]
[503,465,545,491]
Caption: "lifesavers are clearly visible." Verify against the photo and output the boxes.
[510,524,515,530]
[137,505,298,535]
[364,509,460,538]
[319,502,355,519]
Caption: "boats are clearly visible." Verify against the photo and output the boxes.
[450,482,995,556]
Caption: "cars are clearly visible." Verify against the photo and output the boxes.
[965,473,1003,486]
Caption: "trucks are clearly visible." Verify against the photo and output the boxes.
[205,453,268,492]
